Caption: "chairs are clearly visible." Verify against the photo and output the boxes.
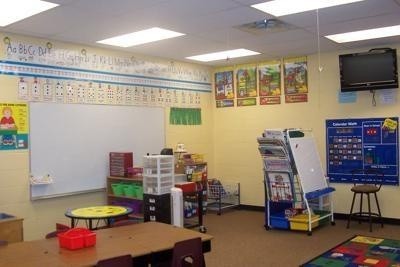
[96,236,206,267]
[345,169,385,232]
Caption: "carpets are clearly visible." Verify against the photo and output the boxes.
[297,234,400,267]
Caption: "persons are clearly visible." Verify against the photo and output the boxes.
[0,107,17,130]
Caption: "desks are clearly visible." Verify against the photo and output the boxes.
[65,205,133,229]
[0,219,214,266]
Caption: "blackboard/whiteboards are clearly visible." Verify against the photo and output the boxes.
[29,100,165,201]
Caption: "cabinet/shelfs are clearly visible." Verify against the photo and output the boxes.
[105,175,143,225]
[142,154,174,223]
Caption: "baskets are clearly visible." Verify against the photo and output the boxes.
[112,201,141,213]
[111,182,143,199]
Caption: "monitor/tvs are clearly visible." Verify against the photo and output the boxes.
[338,49,398,92]
